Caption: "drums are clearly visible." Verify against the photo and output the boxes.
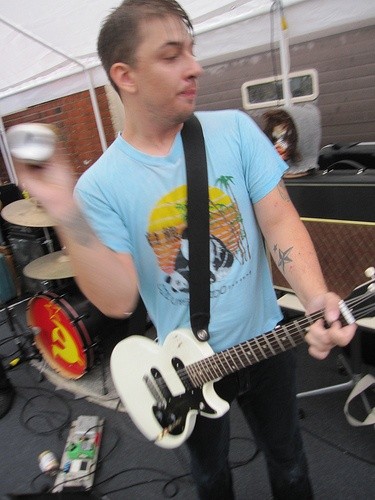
[25,289,99,383]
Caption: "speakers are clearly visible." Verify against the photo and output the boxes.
[268,168,375,298]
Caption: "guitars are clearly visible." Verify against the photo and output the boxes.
[109,265,375,452]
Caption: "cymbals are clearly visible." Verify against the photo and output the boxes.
[21,250,76,280]
[1,198,59,227]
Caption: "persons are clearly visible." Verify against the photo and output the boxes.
[10,0,362,500]
